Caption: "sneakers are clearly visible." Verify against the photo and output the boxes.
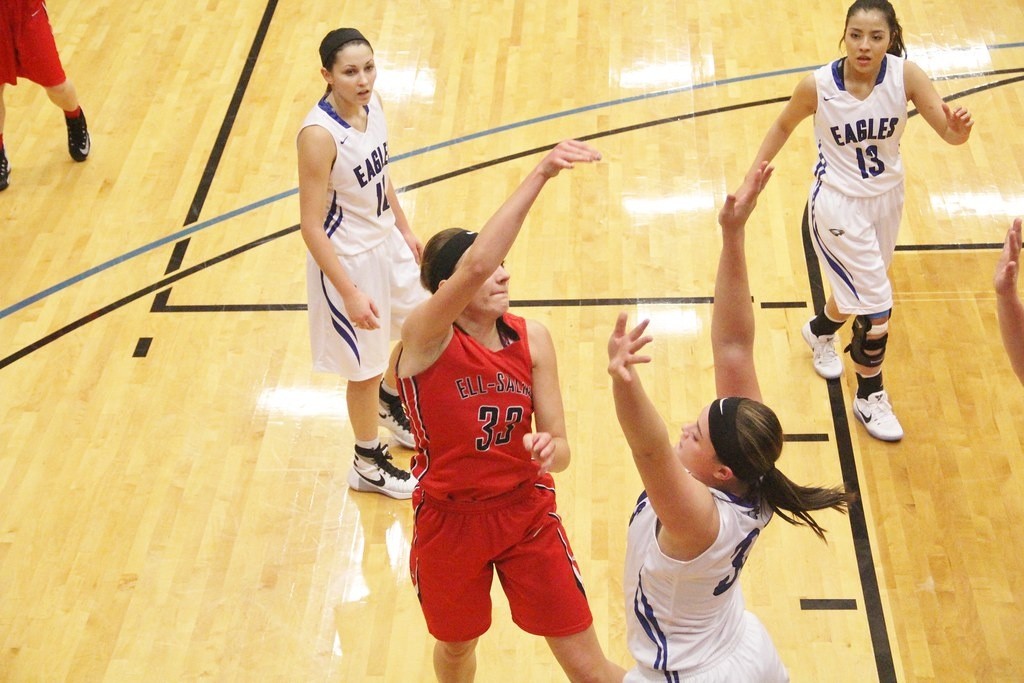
[801,316,844,378]
[64,108,89,162]
[853,388,904,441]
[377,378,417,451]
[348,445,418,499]
[0,150,11,190]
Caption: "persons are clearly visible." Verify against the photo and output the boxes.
[395,142,630,683]
[610,163,856,683]
[996,216,1023,385]
[294,29,423,500]
[735,1,973,441]
[2,2,89,190]
